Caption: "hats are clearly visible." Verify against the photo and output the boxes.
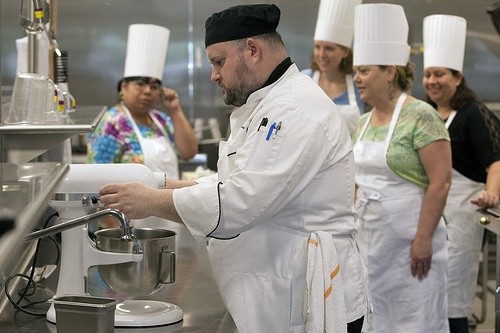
[123,23,170,82]
[204,3,280,47]
[423,14,467,73]
[314,0,362,49]
[352,3,411,67]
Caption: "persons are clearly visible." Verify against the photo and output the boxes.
[350,4,452,333]
[300,0,365,138]
[87,23,198,180]
[421,14,500,333]
[99,3,374,333]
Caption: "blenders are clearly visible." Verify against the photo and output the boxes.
[46,164,184,325]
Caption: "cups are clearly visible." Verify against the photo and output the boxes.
[3,72,64,125]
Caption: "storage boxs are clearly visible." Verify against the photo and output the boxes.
[45,295,124,333]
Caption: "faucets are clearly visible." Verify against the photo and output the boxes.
[24,206,135,243]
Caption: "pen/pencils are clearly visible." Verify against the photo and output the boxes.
[273,121,282,139]
[265,122,277,140]
[256,117,268,132]
